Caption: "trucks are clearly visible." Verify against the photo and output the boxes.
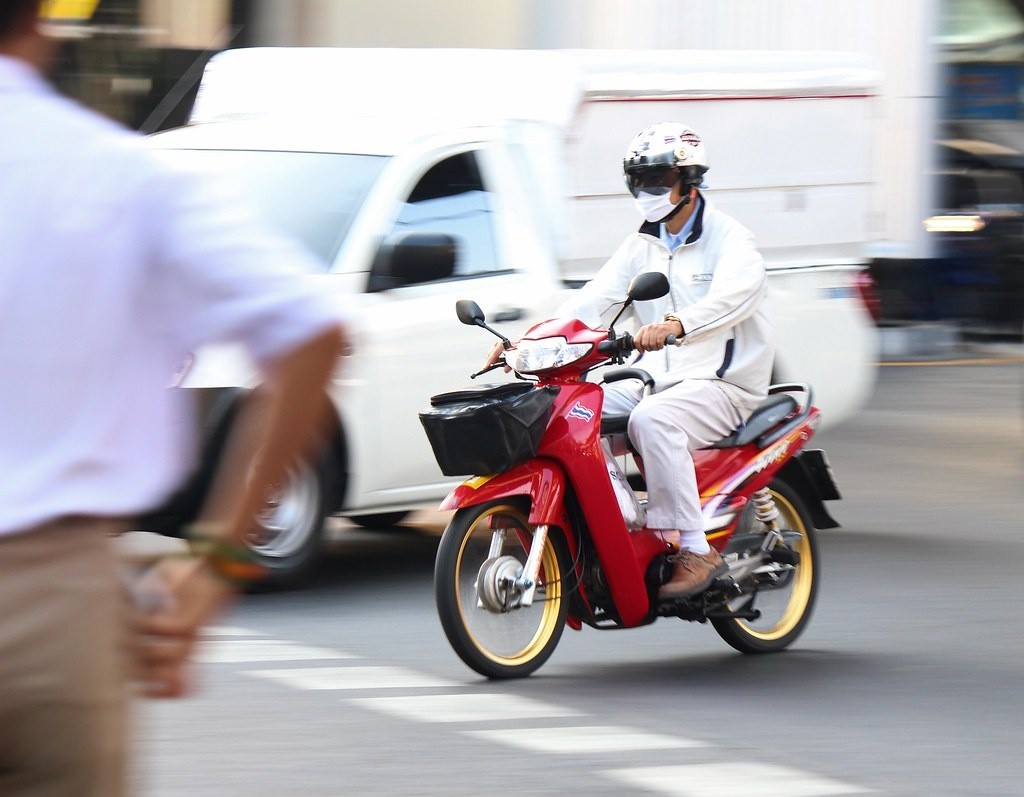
[105,46,884,597]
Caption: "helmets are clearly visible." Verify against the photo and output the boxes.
[622,122,711,199]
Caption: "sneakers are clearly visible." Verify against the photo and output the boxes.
[659,545,729,598]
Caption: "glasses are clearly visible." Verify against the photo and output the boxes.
[626,166,686,198]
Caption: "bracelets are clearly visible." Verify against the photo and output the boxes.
[185,532,273,585]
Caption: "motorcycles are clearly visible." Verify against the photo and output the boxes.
[418,271,843,682]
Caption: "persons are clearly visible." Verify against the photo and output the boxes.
[483,119,777,606]
[2,2,360,797]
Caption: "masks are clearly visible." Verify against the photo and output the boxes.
[634,190,690,222]
